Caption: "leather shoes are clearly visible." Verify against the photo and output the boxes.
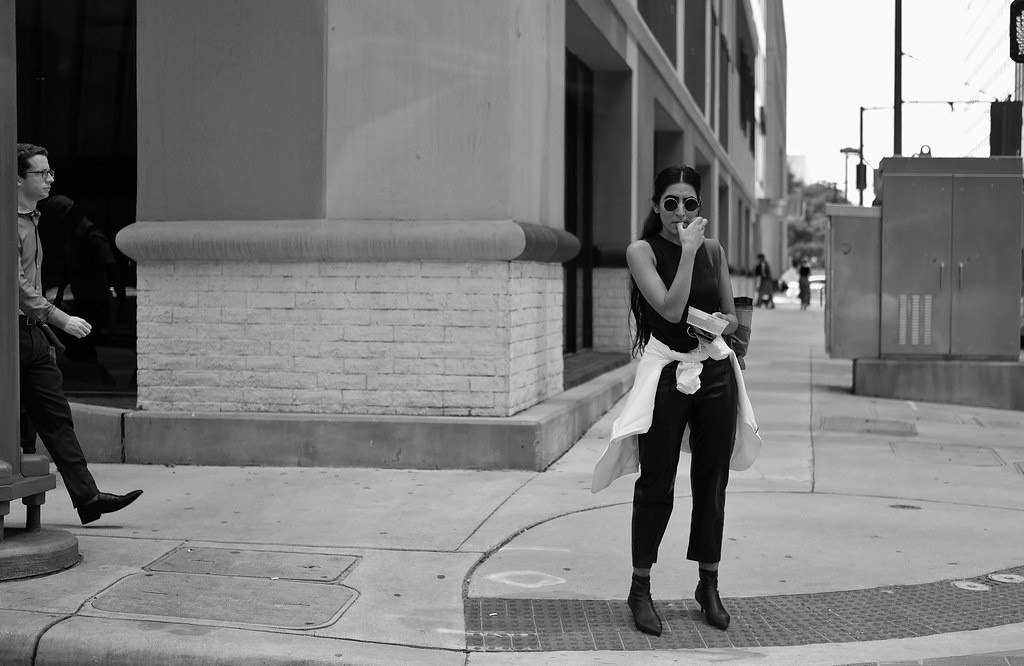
[77,489,143,524]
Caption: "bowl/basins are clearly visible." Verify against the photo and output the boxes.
[687,306,730,336]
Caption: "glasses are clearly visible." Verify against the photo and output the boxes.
[659,196,701,211]
[18,169,54,178]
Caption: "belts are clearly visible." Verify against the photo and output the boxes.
[19,315,42,327]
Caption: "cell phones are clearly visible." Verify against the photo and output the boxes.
[694,327,713,342]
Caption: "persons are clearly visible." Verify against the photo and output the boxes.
[626,166,764,637]
[797,260,810,310]
[756,254,775,309]
[18,142,143,524]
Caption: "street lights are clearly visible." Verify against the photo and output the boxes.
[841,147,859,203]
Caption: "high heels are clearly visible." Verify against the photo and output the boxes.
[628,572,662,636]
[695,568,730,630]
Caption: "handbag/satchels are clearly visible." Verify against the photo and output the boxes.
[705,239,754,370]
